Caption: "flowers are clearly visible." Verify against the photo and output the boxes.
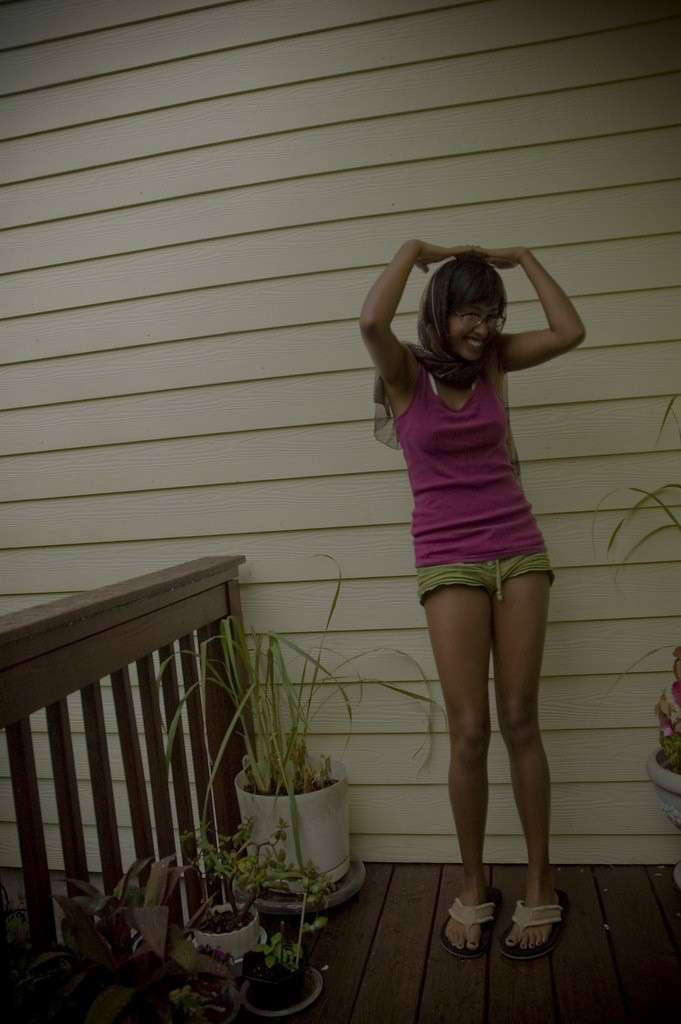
[588,394,681,780]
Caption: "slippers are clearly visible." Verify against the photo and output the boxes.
[439,887,570,959]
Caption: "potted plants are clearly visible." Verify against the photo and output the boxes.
[179,817,336,965]
[27,854,241,1024]
[157,554,448,913]
[241,922,324,1018]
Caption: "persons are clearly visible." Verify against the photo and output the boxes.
[358,238,588,960]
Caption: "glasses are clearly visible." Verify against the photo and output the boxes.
[454,312,506,329]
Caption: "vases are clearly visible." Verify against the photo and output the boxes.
[646,745,681,888]
